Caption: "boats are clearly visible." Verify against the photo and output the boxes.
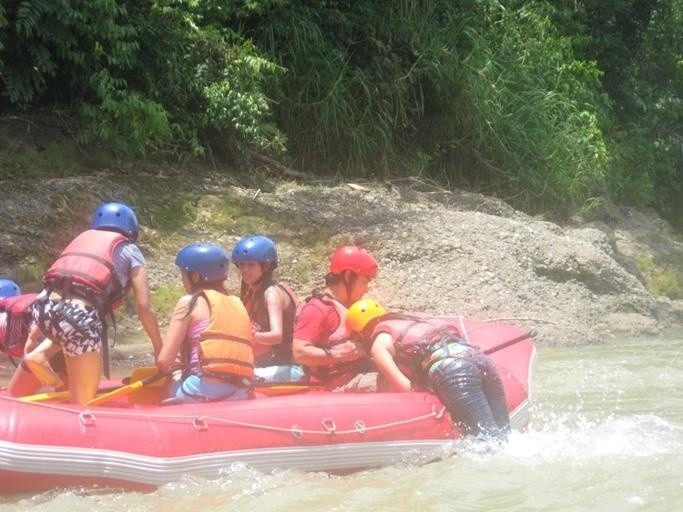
[0,316,536,496]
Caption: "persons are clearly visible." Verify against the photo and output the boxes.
[157,243,253,404]
[23,202,163,407]
[292,245,382,395]
[231,235,303,383]
[344,298,512,447]
[0,276,69,397]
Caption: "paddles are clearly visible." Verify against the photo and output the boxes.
[18,390,67,405]
[87,369,169,407]
[257,380,320,396]
[131,367,166,405]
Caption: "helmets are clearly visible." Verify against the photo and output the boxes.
[174,242,230,281]
[329,245,380,281]
[92,201,139,243]
[231,235,279,263]
[0,277,21,299]
[344,299,387,336]
